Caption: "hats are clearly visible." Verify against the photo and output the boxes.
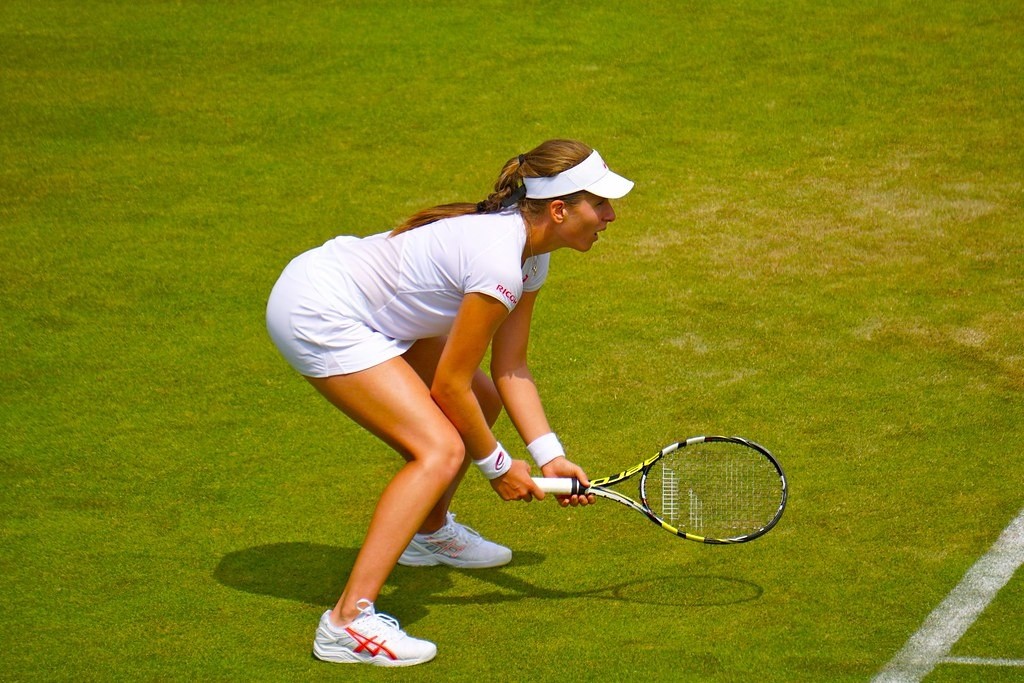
[523,149,635,199]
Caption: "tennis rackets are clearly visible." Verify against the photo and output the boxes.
[529,434,790,546]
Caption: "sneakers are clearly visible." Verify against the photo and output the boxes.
[312,599,437,668]
[395,511,515,568]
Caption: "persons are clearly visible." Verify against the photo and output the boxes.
[266,138,634,670]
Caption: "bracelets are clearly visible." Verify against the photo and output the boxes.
[472,441,512,480]
[527,432,565,470]
